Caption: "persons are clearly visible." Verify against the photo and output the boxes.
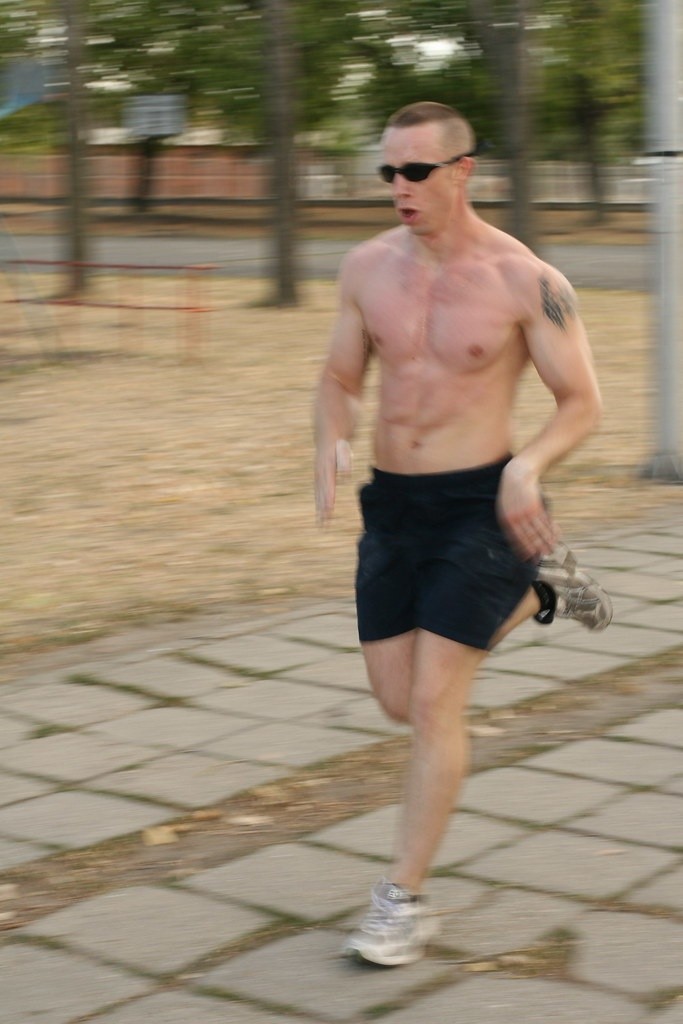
[311,100,614,967]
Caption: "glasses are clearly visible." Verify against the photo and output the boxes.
[379,150,477,183]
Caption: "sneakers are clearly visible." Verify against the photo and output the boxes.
[345,875,437,966]
[534,541,612,633]
